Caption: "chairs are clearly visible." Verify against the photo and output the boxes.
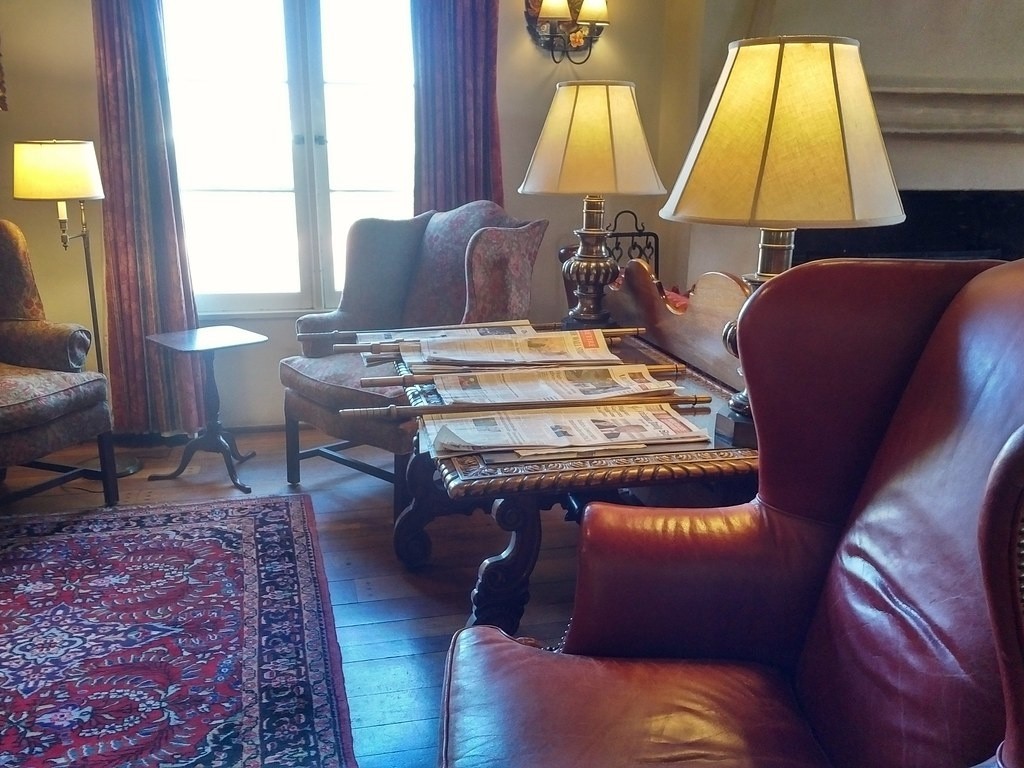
[0,219,119,505]
[279,200,549,527]
[442,258,1024,768]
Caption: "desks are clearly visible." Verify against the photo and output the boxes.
[148,325,268,493]
[393,260,762,636]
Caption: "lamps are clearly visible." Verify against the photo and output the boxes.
[516,80,670,330]
[660,35,907,448]
[524,0,610,65]
[14,140,143,477]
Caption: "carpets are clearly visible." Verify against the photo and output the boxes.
[0,493,359,768]
